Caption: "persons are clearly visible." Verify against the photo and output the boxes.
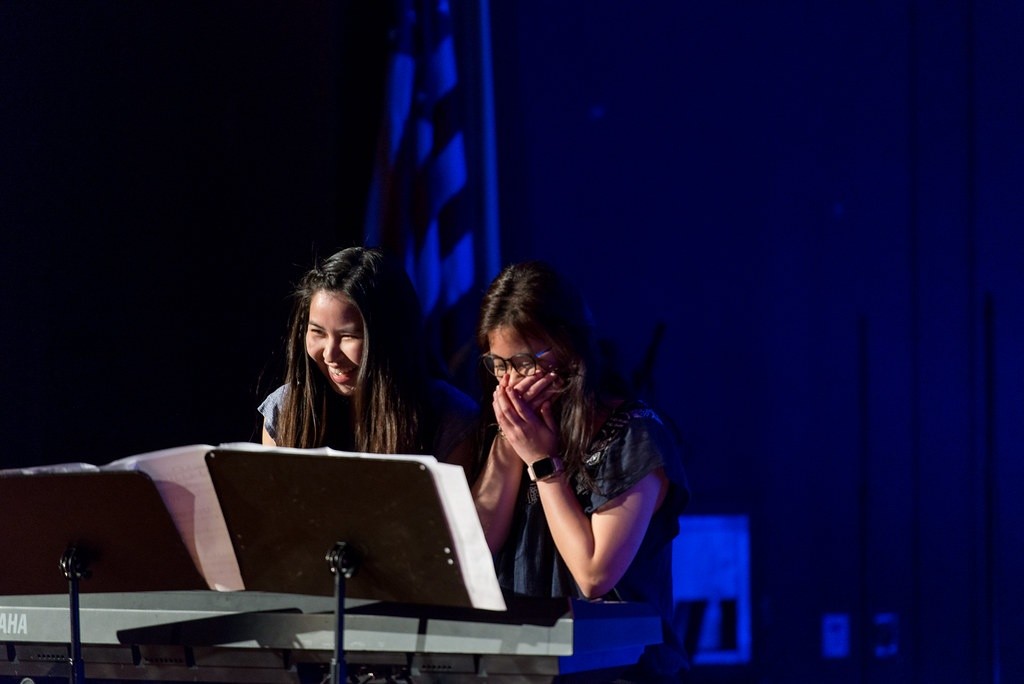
[472,262,688,684]
[256,243,483,486]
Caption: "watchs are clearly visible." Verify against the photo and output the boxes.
[527,455,565,481]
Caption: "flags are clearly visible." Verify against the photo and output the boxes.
[372,0,505,462]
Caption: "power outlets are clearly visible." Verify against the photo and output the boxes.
[874,612,898,656]
[823,615,849,657]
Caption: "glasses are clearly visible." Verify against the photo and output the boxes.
[483,351,545,377]
